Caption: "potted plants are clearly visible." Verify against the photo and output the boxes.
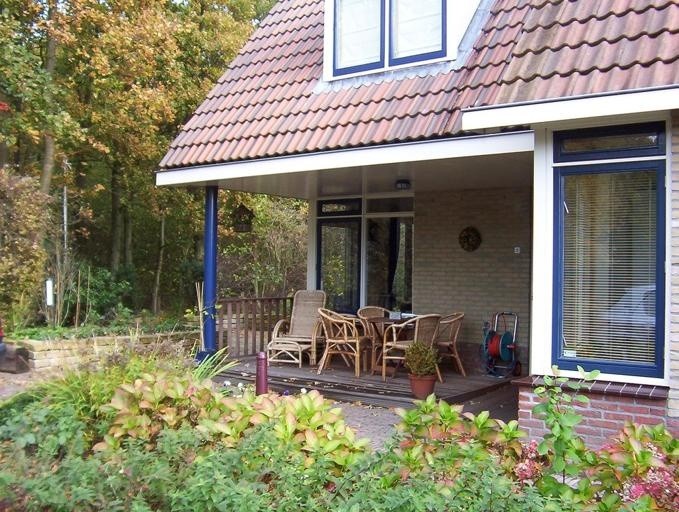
[400,338,444,403]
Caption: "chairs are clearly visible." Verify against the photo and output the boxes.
[265,289,467,381]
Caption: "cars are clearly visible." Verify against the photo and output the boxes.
[590,284,655,362]
